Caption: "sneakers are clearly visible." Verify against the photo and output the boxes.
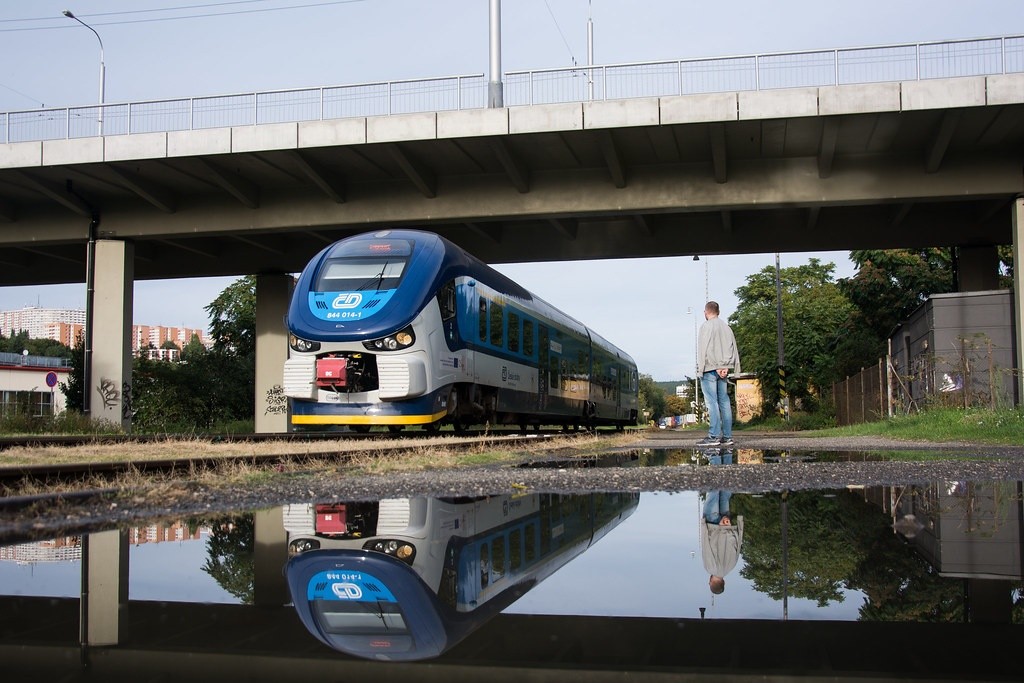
[721,448,733,455]
[696,448,720,460]
[720,437,735,445]
[696,437,721,445]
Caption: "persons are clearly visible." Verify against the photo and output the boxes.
[699,446,744,595]
[695,302,741,446]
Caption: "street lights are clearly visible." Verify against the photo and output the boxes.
[63,10,105,137]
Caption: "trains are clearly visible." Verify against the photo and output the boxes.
[282,228,638,432]
[281,490,642,662]
